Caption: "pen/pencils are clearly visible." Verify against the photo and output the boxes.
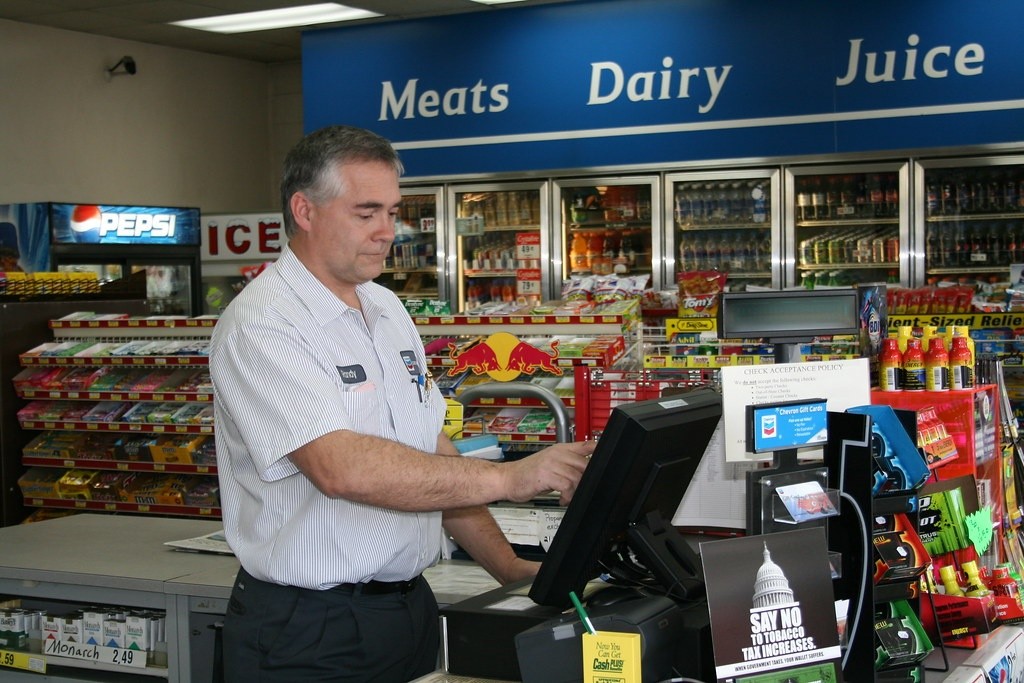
[569,592,596,634]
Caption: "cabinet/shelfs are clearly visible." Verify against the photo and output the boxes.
[870,384,1000,574]
[888,314,1024,439]
[164,556,504,683]
[0,512,237,683]
[402,300,641,453]
[12,311,222,515]
[590,321,877,443]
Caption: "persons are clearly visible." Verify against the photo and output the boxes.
[208,125,597,683]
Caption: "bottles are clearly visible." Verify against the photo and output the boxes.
[675,179,772,273]
[938,560,1024,616]
[795,173,901,290]
[923,178,1024,290]
[454,190,545,305]
[565,192,651,274]
[876,336,975,393]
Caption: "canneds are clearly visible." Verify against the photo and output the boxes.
[384,233,438,268]
[472,239,538,269]
[798,228,899,263]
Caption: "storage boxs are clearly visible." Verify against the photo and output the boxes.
[26,611,39,631]
[0,609,25,649]
[103,618,126,649]
[125,614,151,651]
[152,615,164,641]
[104,611,116,619]
[83,609,108,646]
[113,612,123,619]
[41,614,62,653]
[150,617,159,651]
[112,608,131,617]
[60,616,83,644]
[24,612,32,633]
[17,312,220,510]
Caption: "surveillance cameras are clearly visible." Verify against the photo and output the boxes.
[123,57,137,74]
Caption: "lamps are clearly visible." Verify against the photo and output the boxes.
[104,54,140,84]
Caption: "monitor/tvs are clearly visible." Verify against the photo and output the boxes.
[529,389,723,611]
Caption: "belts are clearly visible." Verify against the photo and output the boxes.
[331,573,422,594]
